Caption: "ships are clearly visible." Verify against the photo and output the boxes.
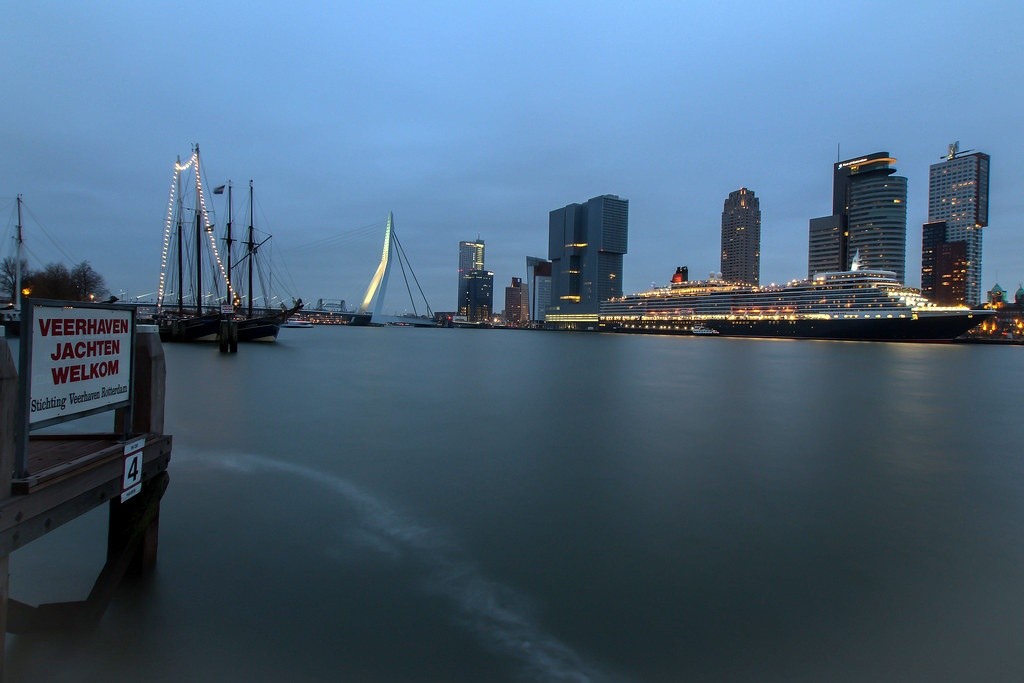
[595,264,998,342]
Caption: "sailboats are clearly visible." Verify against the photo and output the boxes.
[126,140,314,354]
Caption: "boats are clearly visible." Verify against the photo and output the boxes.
[692,322,720,336]
